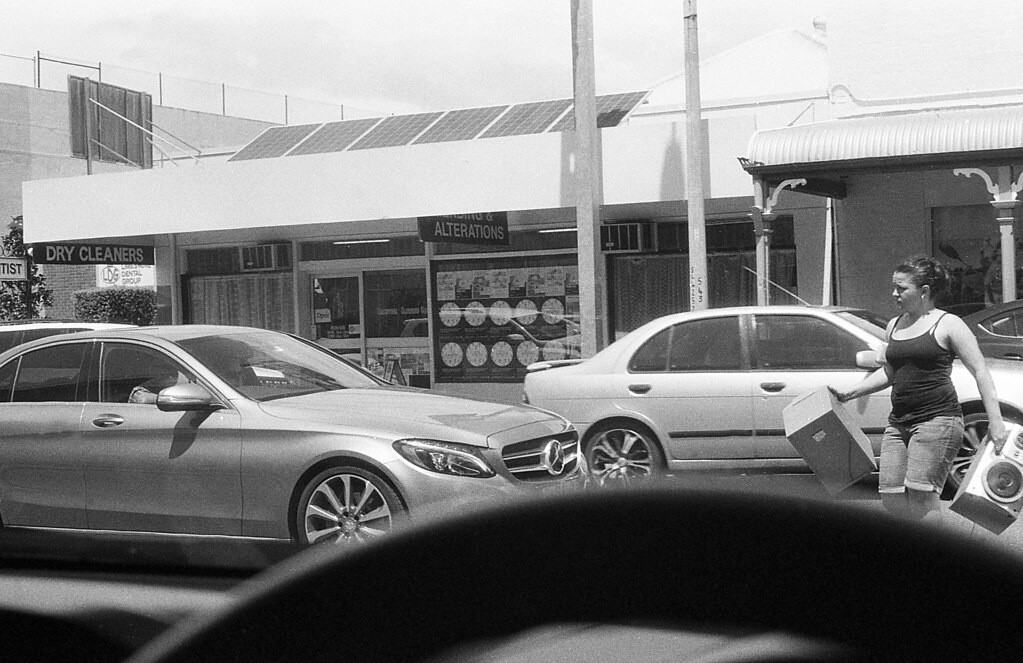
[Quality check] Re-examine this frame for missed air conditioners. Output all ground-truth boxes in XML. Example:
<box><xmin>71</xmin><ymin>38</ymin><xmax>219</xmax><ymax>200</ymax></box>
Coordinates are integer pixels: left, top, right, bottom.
<box><xmin>238</xmin><ymin>242</ymin><xmax>292</xmax><ymax>273</ymax></box>
<box><xmin>599</xmin><ymin>222</ymin><xmax>660</xmax><ymax>255</ymax></box>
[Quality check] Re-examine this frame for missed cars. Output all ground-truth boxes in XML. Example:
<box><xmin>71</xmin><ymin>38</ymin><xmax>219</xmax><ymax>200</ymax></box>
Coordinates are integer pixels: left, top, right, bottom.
<box><xmin>0</xmin><ymin>326</ymin><xmax>589</xmax><ymax>549</ymax></box>
<box><xmin>521</xmin><ymin>303</ymin><xmax>1023</xmax><ymax>494</ymax></box>
<box><xmin>960</xmin><ymin>300</ymin><xmax>1023</xmax><ymax>360</ymax></box>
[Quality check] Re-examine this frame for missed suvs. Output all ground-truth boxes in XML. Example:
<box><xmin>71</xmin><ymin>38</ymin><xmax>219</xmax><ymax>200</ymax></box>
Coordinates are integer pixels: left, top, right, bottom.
<box><xmin>0</xmin><ymin>318</ymin><xmax>292</xmax><ymax>390</ymax></box>
<box><xmin>402</xmin><ymin>307</ymin><xmax>581</xmax><ymax>359</ymax></box>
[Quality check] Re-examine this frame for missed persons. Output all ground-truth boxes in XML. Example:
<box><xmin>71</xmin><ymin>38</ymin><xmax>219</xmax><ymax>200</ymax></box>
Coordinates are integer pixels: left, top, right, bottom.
<box><xmin>127</xmin><ymin>355</ymin><xmax>195</xmax><ymax>403</ymax></box>
<box><xmin>827</xmin><ymin>254</ymin><xmax>1007</xmax><ymax>526</ymax></box>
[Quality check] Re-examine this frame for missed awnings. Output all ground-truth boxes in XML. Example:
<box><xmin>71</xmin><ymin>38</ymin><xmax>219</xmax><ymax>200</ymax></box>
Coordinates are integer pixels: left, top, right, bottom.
<box><xmin>21</xmin><ymin>112</ymin><xmax>758</xmax><ymax>246</ymax></box>
